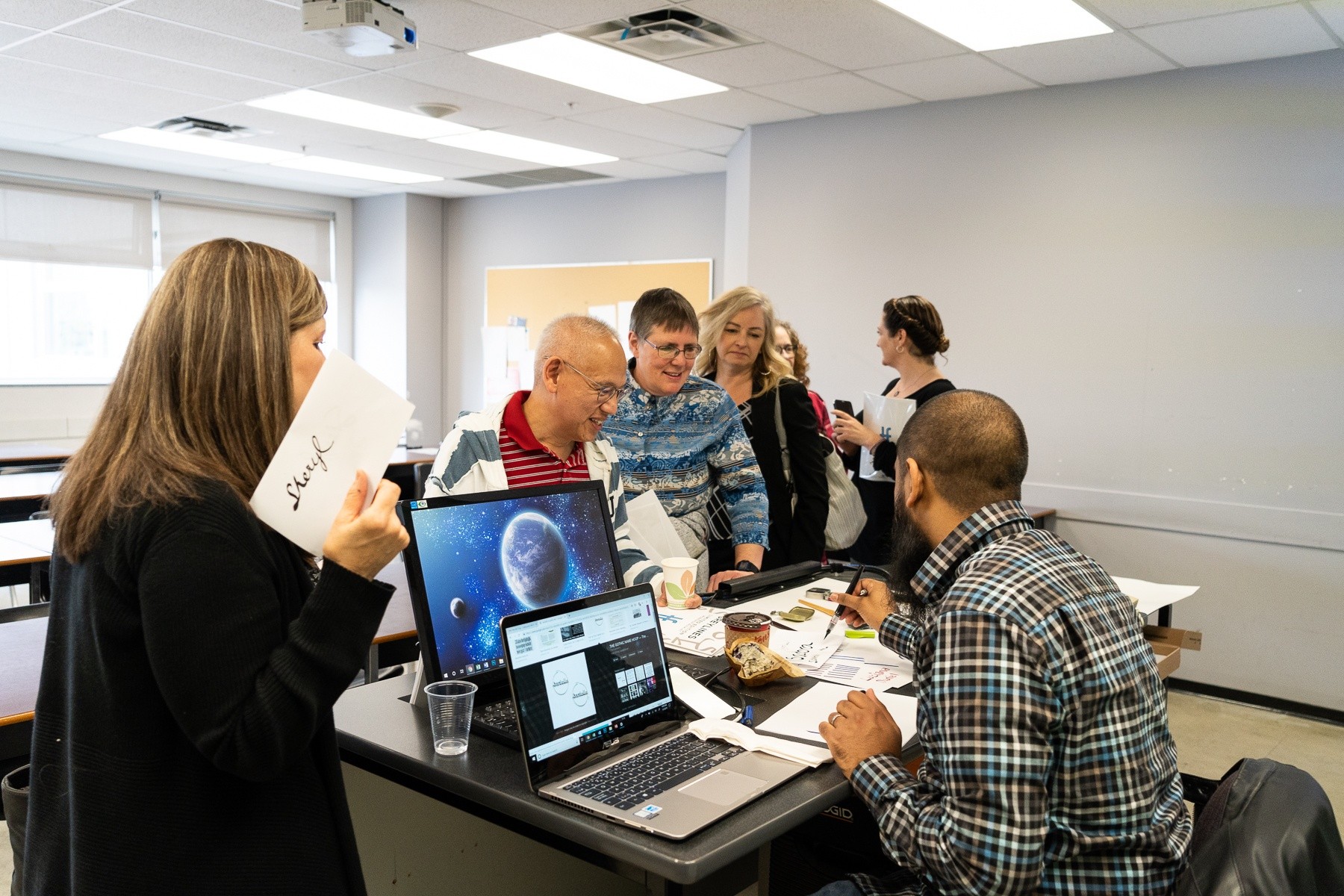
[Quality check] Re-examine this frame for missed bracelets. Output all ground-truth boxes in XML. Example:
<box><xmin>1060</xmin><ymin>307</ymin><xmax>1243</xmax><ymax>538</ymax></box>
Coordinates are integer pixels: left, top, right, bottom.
<box><xmin>869</xmin><ymin>438</ymin><xmax>886</xmax><ymax>454</ymax></box>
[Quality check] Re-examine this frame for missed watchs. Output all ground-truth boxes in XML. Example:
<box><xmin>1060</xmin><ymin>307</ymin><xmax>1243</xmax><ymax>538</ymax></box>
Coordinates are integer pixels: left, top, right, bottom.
<box><xmin>735</xmin><ymin>560</ymin><xmax>760</xmax><ymax>573</ymax></box>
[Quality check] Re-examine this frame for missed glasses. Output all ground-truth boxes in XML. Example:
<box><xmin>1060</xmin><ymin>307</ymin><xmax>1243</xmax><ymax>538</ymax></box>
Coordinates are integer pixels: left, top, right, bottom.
<box><xmin>775</xmin><ymin>344</ymin><xmax>799</xmax><ymax>359</ymax></box>
<box><xmin>543</xmin><ymin>356</ymin><xmax>630</xmax><ymax>404</ymax></box>
<box><xmin>637</xmin><ymin>333</ymin><xmax>704</xmax><ymax>359</ymax></box>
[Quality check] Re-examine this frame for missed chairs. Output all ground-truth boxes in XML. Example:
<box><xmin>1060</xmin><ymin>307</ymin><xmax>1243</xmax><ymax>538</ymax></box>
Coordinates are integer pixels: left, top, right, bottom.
<box><xmin>1173</xmin><ymin>756</ymin><xmax>1344</xmax><ymax>896</ymax></box>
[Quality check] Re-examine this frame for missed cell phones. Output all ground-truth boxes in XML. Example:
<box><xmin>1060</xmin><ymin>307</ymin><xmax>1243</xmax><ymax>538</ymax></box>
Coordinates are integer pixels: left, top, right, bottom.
<box><xmin>834</xmin><ymin>400</ymin><xmax>854</xmax><ymax>427</ymax></box>
<box><xmin>668</xmin><ymin>665</ymin><xmax>742</xmax><ymax>723</ymax></box>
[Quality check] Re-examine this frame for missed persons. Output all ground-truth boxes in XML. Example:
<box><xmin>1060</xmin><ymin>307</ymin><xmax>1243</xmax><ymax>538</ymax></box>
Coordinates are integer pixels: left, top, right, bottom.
<box><xmin>598</xmin><ymin>287</ymin><xmax>771</xmax><ymax>593</ymax></box>
<box><xmin>423</xmin><ymin>316</ymin><xmax>703</xmax><ymax>609</ymax></box>
<box><xmin>831</xmin><ymin>294</ymin><xmax>957</xmax><ymax>566</ymax></box>
<box><xmin>771</xmin><ymin>319</ymin><xmax>838</xmax><ymax>447</ymax></box>
<box><xmin>693</xmin><ymin>286</ymin><xmax>829</xmax><ymax>579</ymax></box>
<box><xmin>20</xmin><ymin>236</ymin><xmax>411</xmax><ymax>896</ymax></box>
<box><xmin>812</xmin><ymin>389</ymin><xmax>1192</xmax><ymax>896</ymax></box>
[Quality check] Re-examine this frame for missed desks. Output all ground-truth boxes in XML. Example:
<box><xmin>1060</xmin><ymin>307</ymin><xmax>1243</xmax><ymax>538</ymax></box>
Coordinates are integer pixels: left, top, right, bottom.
<box><xmin>0</xmin><ymin>449</ymin><xmax>1205</xmax><ymax>896</ymax></box>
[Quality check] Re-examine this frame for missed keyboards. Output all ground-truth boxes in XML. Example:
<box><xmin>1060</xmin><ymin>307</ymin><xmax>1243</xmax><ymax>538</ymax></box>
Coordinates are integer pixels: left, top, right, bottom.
<box><xmin>471</xmin><ymin>658</ymin><xmax>717</xmax><ymax>751</ymax></box>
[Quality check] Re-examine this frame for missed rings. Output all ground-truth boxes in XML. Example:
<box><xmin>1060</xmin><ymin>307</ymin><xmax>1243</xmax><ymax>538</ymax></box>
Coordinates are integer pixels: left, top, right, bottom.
<box><xmin>831</xmin><ymin>714</ymin><xmax>842</xmax><ymax>725</ymax></box>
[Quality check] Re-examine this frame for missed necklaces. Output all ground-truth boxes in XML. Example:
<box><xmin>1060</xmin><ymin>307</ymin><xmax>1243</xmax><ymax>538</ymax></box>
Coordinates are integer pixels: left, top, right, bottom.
<box><xmin>893</xmin><ymin>367</ymin><xmax>936</xmax><ymax>397</ymax></box>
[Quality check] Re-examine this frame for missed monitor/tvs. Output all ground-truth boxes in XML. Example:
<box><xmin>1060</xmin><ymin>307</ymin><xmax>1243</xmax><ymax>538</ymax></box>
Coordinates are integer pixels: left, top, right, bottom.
<box><xmin>395</xmin><ymin>477</ymin><xmax>626</xmax><ymax>706</ymax></box>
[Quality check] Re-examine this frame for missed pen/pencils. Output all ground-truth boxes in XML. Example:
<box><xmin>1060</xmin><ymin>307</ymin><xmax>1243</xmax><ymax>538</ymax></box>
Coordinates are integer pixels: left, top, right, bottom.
<box><xmin>823</xmin><ymin>563</ymin><xmax>866</xmax><ymax>640</ymax></box>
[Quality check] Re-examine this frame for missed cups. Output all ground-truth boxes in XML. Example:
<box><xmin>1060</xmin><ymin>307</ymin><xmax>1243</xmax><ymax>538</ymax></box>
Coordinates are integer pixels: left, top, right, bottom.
<box><xmin>722</xmin><ymin>613</ymin><xmax>771</xmax><ymax>669</ymax></box>
<box><xmin>661</xmin><ymin>557</ymin><xmax>699</xmax><ymax>611</ymax></box>
<box><xmin>424</xmin><ymin>681</ymin><xmax>478</xmax><ymax>755</ymax></box>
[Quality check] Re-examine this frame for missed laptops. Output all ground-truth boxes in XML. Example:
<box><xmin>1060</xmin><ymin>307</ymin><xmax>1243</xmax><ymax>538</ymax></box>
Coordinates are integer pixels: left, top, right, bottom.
<box><xmin>499</xmin><ymin>580</ymin><xmax>810</xmax><ymax>840</ymax></box>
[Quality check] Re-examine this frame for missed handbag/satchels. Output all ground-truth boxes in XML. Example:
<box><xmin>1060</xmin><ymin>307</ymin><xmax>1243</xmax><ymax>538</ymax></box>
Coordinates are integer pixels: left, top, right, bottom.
<box><xmin>818</xmin><ymin>433</ymin><xmax>867</xmax><ymax>549</ymax></box>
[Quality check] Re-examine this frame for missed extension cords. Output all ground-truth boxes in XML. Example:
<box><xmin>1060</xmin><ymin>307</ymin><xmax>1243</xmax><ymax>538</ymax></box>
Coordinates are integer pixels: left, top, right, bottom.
<box><xmin>718</xmin><ymin>561</ymin><xmax>821</xmax><ymax>595</ymax></box>
